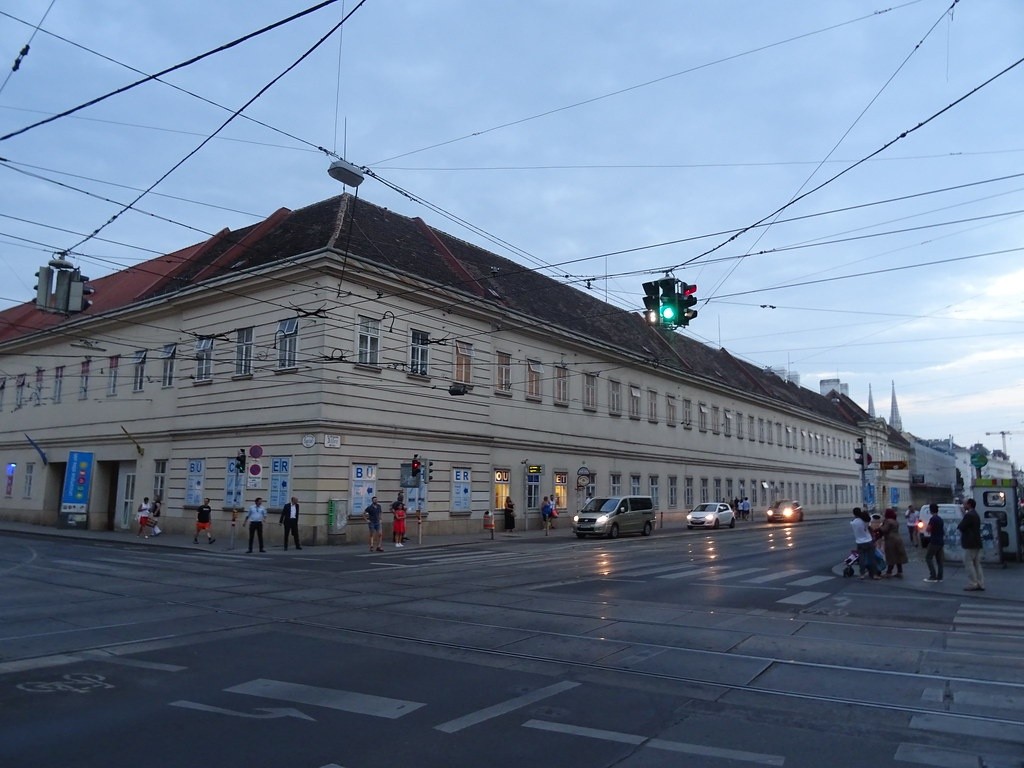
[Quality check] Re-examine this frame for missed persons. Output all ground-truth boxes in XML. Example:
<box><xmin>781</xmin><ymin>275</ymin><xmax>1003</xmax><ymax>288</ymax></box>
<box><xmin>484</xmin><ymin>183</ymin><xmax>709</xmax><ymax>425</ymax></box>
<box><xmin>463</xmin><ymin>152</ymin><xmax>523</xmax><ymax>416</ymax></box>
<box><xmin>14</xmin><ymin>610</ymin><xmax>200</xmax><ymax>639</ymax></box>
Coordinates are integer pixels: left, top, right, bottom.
<box><xmin>851</xmin><ymin>507</ymin><xmax>908</xmax><ymax>579</ymax></box>
<box><xmin>958</xmin><ymin>499</ymin><xmax>984</xmax><ymax>590</ymax></box>
<box><xmin>363</xmin><ymin>496</ymin><xmax>384</xmax><ymax>551</ymax></box>
<box><xmin>243</xmin><ymin>498</ymin><xmax>266</xmax><ymax>553</ymax></box>
<box><xmin>389</xmin><ymin>492</ymin><xmax>410</xmax><ymax>547</ymax></box>
<box><xmin>193</xmin><ymin>498</ymin><xmax>216</xmax><ymax>544</ymax></box>
<box><xmin>722</xmin><ymin>497</ymin><xmax>750</xmax><ymax>521</ymax></box>
<box><xmin>585</xmin><ymin>493</ymin><xmax>592</xmax><ymax>507</ymax></box>
<box><xmin>924</xmin><ymin>503</ymin><xmax>944</xmax><ymax>582</ymax></box>
<box><xmin>541</xmin><ymin>495</ymin><xmax>556</xmax><ymax>530</ymax></box>
<box><xmin>505</xmin><ymin>497</ymin><xmax>515</xmax><ymax>532</ymax></box>
<box><xmin>279</xmin><ymin>496</ymin><xmax>302</xmax><ymax>550</ymax></box>
<box><xmin>905</xmin><ymin>505</ymin><xmax>919</xmax><ymax>547</ymax></box>
<box><xmin>136</xmin><ymin>496</ymin><xmax>162</xmax><ymax>538</ymax></box>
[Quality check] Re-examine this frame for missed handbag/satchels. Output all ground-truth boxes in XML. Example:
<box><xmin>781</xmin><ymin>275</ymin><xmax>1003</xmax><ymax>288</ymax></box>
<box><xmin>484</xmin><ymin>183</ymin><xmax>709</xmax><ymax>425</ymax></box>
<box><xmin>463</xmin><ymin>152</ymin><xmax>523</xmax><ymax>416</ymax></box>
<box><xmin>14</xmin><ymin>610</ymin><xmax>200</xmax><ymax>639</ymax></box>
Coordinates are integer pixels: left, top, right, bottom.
<box><xmin>135</xmin><ymin>509</ymin><xmax>141</xmax><ymax>524</ymax></box>
<box><xmin>511</xmin><ymin>505</ymin><xmax>518</xmax><ymax>517</ymax></box>
<box><xmin>147</xmin><ymin>516</ymin><xmax>158</xmax><ymax>528</ymax></box>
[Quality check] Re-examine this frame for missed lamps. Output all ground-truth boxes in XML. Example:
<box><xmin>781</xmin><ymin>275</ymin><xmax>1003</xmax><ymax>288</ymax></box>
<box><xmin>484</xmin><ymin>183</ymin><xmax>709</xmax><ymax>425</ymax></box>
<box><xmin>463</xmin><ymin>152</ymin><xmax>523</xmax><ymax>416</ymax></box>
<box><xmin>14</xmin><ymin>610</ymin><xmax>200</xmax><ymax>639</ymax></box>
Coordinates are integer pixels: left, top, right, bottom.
<box><xmin>447</xmin><ymin>384</ymin><xmax>467</xmax><ymax>395</ymax></box>
<box><xmin>328</xmin><ymin>161</ymin><xmax>365</xmax><ymax>187</ymax></box>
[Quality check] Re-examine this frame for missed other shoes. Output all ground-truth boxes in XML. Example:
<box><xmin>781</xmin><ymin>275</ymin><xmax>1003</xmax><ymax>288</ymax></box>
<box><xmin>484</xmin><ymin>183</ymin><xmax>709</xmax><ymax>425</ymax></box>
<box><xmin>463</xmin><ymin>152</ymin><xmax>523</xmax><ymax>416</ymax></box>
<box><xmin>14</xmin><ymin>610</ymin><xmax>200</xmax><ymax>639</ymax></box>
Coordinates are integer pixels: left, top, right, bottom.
<box><xmin>395</xmin><ymin>543</ymin><xmax>401</xmax><ymax>547</ymax></box>
<box><xmin>893</xmin><ymin>572</ymin><xmax>902</xmax><ymax>578</ymax></box>
<box><xmin>284</xmin><ymin>547</ymin><xmax>302</xmax><ymax>551</ymax></box>
<box><xmin>209</xmin><ymin>539</ymin><xmax>215</xmax><ymax>544</ymax></box>
<box><xmin>399</xmin><ymin>543</ymin><xmax>403</xmax><ymax>547</ymax></box>
<box><xmin>192</xmin><ymin>538</ymin><xmax>198</xmax><ymax>544</ymax></box>
<box><xmin>937</xmin><ymin>575</ymin><xmax>944</xmax><ymax>582</ymax></box>
<box><xmin>964</xmin><ymin>583</ymin><xmax>979</xmax><ymax>591</ymax></box>
<box><xmin>861</xmin><ymin>574</ymin><xmax>866</xmax><ymax>579</ymax></box>
<box><xmin>874</xmin><ymin>576</ymin><xmax>881</xmax><ymax>580</ymax></box>
<box><xmin>136</xmin><ymin>532</ymin><xmax>162</xmax><ymax>539</ymax></box>
<box><xmin>923</xmin><ymin>576</ymin><xmax>938</xmax><ymax>582</ymax></box>
<box><xmin>978</xmin><ymin>586</ymin><xmax>985</xmax><ymax>590</ymax></box>
<box><xmin>370</xmin><ymin>546</ymin><xmax>374</xmax><ymax>552</ymax></box>
<box><xmin>259</xmin><ymin>550</ymin><xmax>266</xmax><ymax>552</ymax></box>
<box><xmin>376</xmin><ymin>547</ymin><xmax>383</xmax><ymax>551</ymax></box>
<box><xmin>245</xmin><ymin>551</ymin><xmax>252</xmax><ymax>553</ymax></box>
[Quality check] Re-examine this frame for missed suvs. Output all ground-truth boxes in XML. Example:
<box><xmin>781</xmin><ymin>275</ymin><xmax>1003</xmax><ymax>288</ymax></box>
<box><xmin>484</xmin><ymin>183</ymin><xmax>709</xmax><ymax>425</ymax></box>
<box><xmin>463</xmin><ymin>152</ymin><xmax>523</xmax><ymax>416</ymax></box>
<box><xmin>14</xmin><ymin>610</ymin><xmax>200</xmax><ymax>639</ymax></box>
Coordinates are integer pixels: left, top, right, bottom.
<box><xmin>571</xmin><ymin>496</ymin><xmax>656</xmax><ymax>540</ymax></box>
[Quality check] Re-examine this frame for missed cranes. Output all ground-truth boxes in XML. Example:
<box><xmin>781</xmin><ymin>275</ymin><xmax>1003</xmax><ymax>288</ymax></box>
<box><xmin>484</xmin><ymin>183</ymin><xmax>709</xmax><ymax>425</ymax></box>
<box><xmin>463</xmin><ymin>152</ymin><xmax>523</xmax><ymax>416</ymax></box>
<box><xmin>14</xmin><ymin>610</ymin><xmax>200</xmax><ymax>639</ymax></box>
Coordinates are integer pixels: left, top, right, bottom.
<box><xmin>985</xmin><ymin>430</ymin><xmax>1024</xmax><ymax>462</ymax></box>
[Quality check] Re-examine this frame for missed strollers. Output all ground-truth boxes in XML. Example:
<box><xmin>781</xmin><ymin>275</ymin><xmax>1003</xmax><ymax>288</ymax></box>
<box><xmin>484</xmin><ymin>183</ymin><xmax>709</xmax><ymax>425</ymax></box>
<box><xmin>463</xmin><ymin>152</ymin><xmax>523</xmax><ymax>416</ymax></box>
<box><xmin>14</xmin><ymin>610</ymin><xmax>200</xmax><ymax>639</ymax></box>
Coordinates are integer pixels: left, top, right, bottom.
<box><xmin>841</xmin><ymin>525</ymin><xmax>885</xmax><ymax>579</ymax></box>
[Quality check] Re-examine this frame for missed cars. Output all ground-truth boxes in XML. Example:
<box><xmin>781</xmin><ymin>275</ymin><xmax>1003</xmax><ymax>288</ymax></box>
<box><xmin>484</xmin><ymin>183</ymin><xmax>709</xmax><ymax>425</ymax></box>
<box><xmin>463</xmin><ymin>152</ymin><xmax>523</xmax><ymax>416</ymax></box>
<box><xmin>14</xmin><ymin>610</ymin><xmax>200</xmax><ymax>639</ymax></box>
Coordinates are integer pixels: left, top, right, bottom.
<box><xmin>766</xmin><ymin>498</ymin><xmax>804</xmax><ymax>524</ymax></box>
<box><xmin>914</xmin><ymin>502</ymin><xmax>968</xmax><ymax>549</ymax></box>
<box><xmin>685</xmin><ymin>502</ymin><xmax>737</xmax><ymax>530</ymax></box>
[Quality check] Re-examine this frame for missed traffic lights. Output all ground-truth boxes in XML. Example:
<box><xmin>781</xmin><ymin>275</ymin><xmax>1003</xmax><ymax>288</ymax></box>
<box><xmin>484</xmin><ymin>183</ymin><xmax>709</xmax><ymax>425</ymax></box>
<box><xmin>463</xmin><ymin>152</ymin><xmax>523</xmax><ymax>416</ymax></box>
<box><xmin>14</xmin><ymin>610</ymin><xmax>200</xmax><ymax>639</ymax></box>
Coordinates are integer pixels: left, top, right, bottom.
<box><xmin>67</xmin><ymin>269</ymin><xmax>95</xmax><ymax>314</ymax></box>
<box><xmin>677</xmin><ymin>281</ymin><xmax>698</xmax><ymax>327</ymax></box>
<box><xmin>32</xmin><ymin>266</ymin><xmax>55</xmax><ymax>312</ymax></box>
<box><xmin>411</xmin><ymin>458</ymin><xmax>421</xmax><ymax>477</ymax></box>
<box><xmin>642</xmin><ymin>280</ymin><xmax>661</xmax><ymax>326</ymax></box>
<box><xmin>235</xmin><ymin>454</ymin><xmax>246</xmax><ymax>473</ymax></box>
<box><xmin>854</xmin><ymin>444</ymin><xmax>863</xmax><ymax>465</ymax></box>
<box><xmin>424</xmin><ymin>459</ymin><xmax>433</xmax><ymax>484</ymax></box>
<box><xmin>657</xmin><ymin>277</ymin><xmax>677</xmax><ymax>328</ymax></box>
